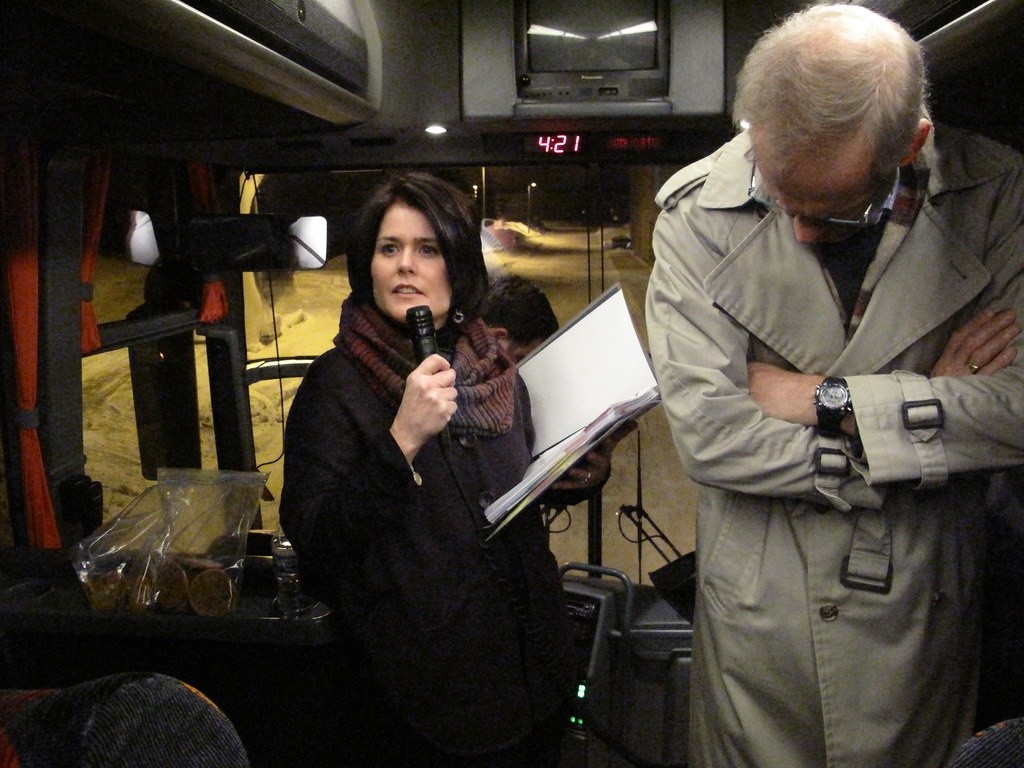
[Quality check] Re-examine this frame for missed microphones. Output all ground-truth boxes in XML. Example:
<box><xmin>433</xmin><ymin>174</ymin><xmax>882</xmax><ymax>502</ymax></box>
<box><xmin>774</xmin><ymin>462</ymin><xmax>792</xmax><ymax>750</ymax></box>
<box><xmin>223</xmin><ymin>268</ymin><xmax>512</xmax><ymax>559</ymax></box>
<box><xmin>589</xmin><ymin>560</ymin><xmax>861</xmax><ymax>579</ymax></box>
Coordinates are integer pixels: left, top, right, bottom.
<box><xmin>407</xmin><ymin>306</ymin><xmax>452</xmax><ymax>451</ymax></box>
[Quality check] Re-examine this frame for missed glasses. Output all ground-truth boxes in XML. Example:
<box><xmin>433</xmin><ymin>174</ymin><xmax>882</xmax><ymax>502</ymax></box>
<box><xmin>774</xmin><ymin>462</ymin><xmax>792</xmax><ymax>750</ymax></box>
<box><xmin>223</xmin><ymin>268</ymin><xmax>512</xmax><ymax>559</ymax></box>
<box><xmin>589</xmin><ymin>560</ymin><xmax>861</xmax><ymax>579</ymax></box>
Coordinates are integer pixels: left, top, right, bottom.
<box><xmin>748</xmin><ymin>159</ymin><xmax>900</xmax><ymax>229</ymax></box>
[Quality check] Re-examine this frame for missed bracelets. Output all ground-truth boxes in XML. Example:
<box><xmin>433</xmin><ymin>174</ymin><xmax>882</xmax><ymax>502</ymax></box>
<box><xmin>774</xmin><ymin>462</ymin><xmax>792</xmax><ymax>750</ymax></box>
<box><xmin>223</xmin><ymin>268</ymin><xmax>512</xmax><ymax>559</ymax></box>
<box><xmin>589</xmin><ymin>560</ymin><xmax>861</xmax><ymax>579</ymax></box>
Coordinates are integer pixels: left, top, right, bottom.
<box><xmin>409</xmin><ymin>463</ymin><xmax>423</xmax><ymax>486</ymax></box>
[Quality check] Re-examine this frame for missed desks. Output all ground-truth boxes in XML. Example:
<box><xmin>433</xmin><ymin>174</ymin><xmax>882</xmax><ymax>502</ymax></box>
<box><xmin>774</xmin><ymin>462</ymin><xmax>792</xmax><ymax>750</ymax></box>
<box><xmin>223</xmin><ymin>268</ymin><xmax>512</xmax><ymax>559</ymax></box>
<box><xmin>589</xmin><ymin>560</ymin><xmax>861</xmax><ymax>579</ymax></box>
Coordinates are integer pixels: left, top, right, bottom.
<box><xmin>0</xmin><ymin>553</ymin><xmax>347</xmax><ymax>646</ymax></box>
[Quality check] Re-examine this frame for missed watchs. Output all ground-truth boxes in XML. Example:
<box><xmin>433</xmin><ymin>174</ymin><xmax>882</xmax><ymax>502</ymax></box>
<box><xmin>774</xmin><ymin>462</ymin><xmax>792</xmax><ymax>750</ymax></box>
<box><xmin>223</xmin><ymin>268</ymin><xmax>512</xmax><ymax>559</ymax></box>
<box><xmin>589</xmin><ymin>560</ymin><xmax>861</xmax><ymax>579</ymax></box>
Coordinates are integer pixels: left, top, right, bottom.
<box><xmin>812</xmin><ymin>375</ymin><xmax>853</xmax><ymax>433</ymax></box>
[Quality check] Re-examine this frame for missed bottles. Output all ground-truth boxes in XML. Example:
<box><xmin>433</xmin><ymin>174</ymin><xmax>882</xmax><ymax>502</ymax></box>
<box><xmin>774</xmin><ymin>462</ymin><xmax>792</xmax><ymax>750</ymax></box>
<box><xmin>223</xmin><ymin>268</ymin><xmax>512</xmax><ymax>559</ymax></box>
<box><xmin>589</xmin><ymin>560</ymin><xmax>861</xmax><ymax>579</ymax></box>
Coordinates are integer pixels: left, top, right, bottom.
<box><xmin>271</xmin><ymin>520</ymin><xmax>300</xmax><ymax>613</ymax></box>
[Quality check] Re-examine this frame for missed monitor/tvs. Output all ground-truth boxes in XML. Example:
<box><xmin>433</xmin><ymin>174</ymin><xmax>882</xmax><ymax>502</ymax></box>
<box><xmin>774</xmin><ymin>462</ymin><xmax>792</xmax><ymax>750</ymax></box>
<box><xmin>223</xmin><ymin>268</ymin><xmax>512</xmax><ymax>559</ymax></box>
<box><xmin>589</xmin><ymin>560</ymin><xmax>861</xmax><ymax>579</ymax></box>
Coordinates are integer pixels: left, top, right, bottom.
<box><xmin>512</xmin><ymin>0</ymin><xmax>671</xmax><ymax>101</ymax></box>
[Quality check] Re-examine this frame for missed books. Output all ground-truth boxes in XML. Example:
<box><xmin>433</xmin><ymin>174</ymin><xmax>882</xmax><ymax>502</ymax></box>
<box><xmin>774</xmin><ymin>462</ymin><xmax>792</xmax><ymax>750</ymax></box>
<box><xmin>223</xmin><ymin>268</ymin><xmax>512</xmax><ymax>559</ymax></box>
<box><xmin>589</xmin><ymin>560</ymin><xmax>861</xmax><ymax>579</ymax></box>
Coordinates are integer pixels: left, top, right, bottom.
<box><xmin>483</xmin><ymin>281</ymin><xmax>660</xmax><ymax>544</ymax></box>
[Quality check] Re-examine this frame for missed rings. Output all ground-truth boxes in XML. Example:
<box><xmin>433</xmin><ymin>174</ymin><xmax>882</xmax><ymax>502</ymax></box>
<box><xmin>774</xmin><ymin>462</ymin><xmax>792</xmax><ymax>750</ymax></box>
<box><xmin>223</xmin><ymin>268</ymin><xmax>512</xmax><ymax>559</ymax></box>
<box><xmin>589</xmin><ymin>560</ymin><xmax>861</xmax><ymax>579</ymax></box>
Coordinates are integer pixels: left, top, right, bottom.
<box><xmin>581</xmin><ymin>472</ymin><xmax>591</xmax><ymax>483</ymax></box>
<box><xmin>967</xmin><ymin>362</ymin><xmax>980</xmax><ymax>373</ymax></box>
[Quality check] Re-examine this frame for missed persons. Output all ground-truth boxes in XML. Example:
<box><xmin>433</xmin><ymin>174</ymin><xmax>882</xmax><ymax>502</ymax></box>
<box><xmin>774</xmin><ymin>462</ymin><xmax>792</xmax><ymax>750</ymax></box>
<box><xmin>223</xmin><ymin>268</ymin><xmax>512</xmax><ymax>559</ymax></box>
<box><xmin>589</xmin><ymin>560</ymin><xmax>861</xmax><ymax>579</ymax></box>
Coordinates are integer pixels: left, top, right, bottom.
<box><xmin>274</xmin><ymin>174</ymin><xmax>639</xmax><ymax>768</ymax></box>
<box><xmin>644</xmin><ymin>5</ymin><xmax>1024</xmax><ymax>768</ymax></box>
<box><xmin>478</xmin><ymin>274</ymin><xmax>560</xmax><ymax>367</ymax></box>
